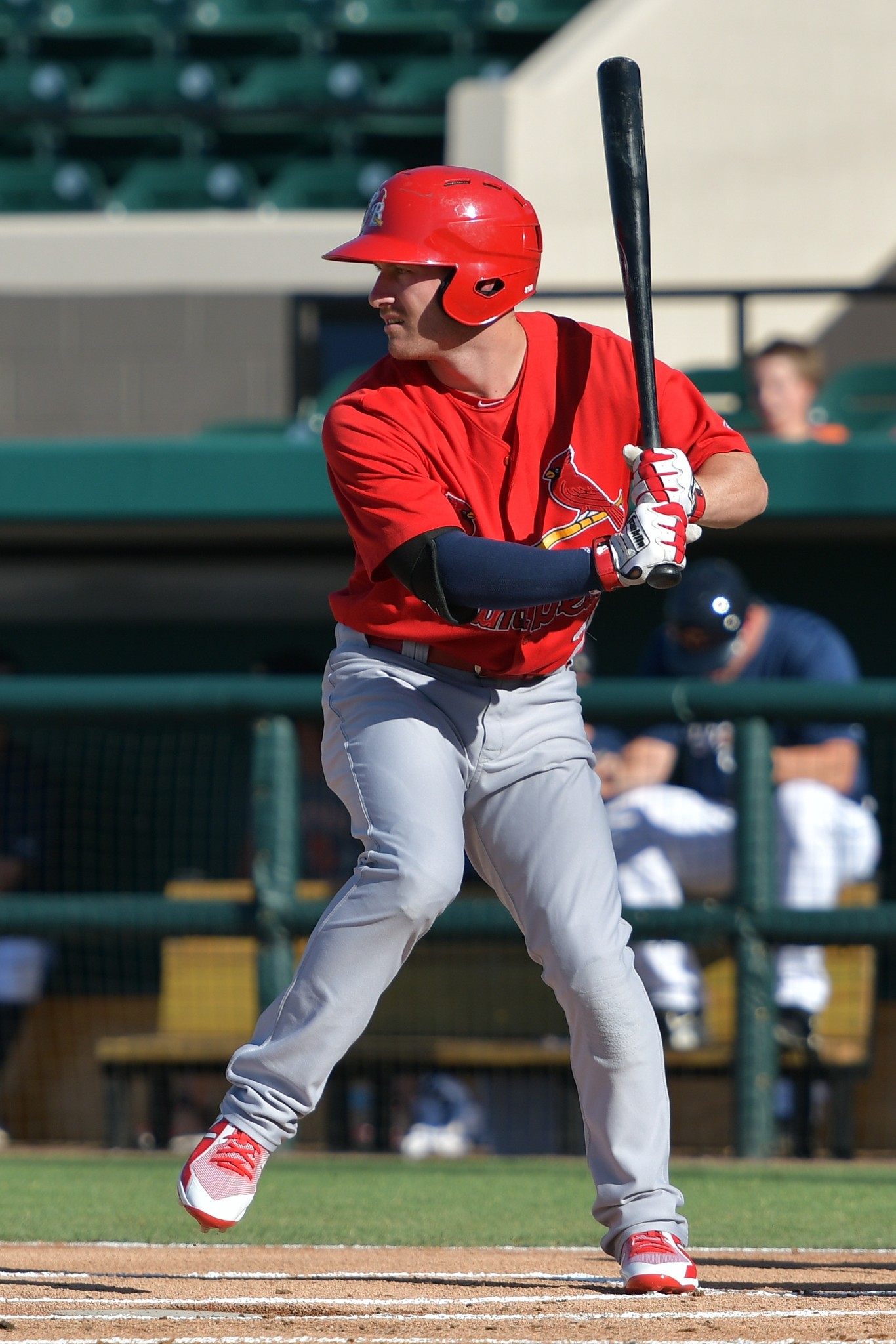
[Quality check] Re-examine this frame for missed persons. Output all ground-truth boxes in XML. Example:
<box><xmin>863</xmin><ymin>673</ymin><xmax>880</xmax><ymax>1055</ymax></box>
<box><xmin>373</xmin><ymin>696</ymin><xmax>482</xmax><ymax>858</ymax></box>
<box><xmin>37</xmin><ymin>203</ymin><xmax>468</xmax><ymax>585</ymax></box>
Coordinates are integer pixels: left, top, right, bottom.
<box><xmin>0</xmin><ymin>840</ymin><xmax>44</xmax><ymax>1144</ymax></box>
<box><xmin>594</xmin><ymin>552</ymin><xmax>881</xmax><ymax>1060</ymax></box>
<box><xmin>180</xmin><ymin>168</ymin><xmax>768</xmax><ymax>1295</ymax></box>
<box><xmin>738</xmin><ymin>339</ymin><xmax>846</xmax><ymax>446</ymax></box>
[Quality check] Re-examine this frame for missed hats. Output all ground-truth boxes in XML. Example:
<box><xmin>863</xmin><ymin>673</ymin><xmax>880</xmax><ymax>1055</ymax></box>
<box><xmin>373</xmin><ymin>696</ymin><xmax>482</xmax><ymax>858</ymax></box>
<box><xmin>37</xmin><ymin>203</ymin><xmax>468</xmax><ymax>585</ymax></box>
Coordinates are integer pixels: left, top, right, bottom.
<box><xmin>661</xmin><ymin>559</ymin><xmax>753</xmax><ymax>676</ymax></box>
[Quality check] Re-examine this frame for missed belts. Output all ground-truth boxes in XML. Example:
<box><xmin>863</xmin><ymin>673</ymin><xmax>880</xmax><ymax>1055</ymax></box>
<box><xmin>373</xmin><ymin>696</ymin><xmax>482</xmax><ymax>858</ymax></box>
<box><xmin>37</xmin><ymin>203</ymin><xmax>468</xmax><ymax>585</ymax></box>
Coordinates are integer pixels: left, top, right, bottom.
<box><xmin>361</xmin><ymin>633</ymin><xmax>539</xmax><ymax>682</ymax></box>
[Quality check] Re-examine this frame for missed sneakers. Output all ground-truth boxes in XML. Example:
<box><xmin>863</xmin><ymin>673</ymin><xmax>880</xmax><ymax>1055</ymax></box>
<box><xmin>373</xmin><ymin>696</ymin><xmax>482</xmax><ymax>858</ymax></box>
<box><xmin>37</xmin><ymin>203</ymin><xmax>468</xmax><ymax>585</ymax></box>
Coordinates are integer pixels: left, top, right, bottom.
<box><xmin>175</xmin><ymin>1113</ymin><xmax>271</xmax><ymax>1232</ymax></box>
<box><xmin>622</xmin><ymin>1231</ymin><xmax>699</xmax><ymax>1294</ymax></box>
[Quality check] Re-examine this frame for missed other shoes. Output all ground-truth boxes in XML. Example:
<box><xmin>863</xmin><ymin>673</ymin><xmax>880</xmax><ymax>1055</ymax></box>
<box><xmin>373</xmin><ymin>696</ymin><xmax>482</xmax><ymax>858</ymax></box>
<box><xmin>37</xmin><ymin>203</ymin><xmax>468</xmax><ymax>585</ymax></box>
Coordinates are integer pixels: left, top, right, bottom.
<box><xmin>656</xmin><ymin>1009</ymin><xmax>706</xmax><ymax>1049</ymax></box>
<box><xmin>773</xmin><ymin>1014</ymin><xmax>813</xmax><ymax>1071</ymax></box>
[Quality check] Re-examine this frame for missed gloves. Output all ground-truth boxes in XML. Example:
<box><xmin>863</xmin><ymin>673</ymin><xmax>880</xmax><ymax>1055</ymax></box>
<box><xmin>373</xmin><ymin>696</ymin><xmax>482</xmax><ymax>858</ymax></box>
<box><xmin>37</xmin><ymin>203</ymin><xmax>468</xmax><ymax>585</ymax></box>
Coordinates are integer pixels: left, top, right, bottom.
<box><xmin>591</xmin><ymin>501</ymin><xmax>702</xmax><ymax>595</ymax></box>
<box><xmin>621</xmin><ymin>444</ymin><xmax>706</xmax><ymax>525</ymax></box>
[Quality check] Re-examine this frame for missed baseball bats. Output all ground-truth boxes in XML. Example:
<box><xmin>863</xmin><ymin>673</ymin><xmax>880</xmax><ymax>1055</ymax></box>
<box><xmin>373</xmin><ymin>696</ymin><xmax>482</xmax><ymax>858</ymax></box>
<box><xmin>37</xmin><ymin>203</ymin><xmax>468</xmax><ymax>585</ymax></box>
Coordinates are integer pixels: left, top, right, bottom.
<box><xmin>598</xmin><ymin>54</ymin><xmax>687</xmax><ymax>589</ymax></box>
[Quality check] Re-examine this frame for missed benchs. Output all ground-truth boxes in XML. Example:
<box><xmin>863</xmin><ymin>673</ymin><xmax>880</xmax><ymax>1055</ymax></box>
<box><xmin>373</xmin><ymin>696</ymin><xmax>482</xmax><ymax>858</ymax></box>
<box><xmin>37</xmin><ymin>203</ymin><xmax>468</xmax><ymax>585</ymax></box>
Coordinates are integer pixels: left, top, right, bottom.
<box><xmin>95</xmin><ymin>877</ymin><xmax>876</xmax><ymax>1159</ymax></box>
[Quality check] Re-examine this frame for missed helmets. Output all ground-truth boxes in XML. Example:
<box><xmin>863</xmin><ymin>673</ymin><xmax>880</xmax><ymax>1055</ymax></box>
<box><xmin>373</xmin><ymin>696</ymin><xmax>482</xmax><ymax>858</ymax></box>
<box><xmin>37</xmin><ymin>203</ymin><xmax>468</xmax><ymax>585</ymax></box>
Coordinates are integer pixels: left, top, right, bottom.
<box><xmin>325</xmin><ymin>166</ymin><xmax>542</xmax><ymax>328</ymax></box>
